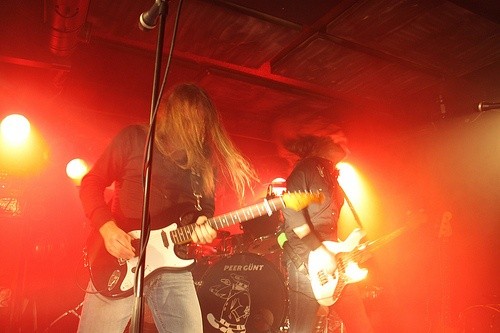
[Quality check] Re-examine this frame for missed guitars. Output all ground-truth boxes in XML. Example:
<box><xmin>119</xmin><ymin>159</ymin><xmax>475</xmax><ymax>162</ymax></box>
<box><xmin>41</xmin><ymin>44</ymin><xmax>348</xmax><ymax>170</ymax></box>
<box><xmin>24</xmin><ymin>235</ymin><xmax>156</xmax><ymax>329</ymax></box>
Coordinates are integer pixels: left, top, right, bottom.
<box><xmin>88</xmin><ymin>189</ymin><xmax>326</xmax><ymax>300</ymax></box>
<box><xmin>308</xmin><ymin>209</ymin><xmax>427</xmax><ymax>307</ymax></box>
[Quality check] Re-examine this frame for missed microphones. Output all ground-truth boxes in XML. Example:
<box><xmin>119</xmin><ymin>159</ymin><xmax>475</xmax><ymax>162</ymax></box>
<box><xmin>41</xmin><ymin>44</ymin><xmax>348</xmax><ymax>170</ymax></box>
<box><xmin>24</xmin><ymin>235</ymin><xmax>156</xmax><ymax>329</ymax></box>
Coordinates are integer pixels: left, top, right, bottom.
<box><xmin>475</xmin><ymin>102</ymin><xmax>500</xmax><ymax>112</ymax></box>
<box><xmin>138</xmin><ymin>0</ymin><xmax>162</xmax><ymax>31</ymax></box>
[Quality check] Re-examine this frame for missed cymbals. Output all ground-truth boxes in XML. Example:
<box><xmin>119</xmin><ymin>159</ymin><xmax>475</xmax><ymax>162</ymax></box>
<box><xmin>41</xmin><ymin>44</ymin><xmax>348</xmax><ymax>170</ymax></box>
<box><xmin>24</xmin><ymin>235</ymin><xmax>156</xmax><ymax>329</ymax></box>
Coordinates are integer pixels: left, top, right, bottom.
<box><xmin>262</xmin><ymin>182</ymin><xmax>287</xmax><ymax>188</ymax></box>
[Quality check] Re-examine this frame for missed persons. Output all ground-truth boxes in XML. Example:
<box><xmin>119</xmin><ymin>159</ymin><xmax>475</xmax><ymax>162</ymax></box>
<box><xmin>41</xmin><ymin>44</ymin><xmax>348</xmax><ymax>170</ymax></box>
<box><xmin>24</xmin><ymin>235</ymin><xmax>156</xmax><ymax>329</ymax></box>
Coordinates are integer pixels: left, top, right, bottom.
<box><xmin>77</xmin><ymin>84</ymin><xmax>261</xmax><ymax>333</ymax></box>
<box><xmin>283</xmin><ymin>135</ymin><xmax>374</xmax><ymax>333</ymax></box>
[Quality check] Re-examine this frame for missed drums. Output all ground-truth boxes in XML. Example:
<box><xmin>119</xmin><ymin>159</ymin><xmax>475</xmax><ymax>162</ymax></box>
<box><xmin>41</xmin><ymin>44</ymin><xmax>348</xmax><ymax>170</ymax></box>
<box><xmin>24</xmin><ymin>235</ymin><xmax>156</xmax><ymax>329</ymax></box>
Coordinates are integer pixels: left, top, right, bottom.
<box><xmin>186</xmin><ymin>230</ymin><xmax>224</xmax><ymax>257</ymax></box>
<box><xmin>239</xmin><ymin>201</ymin><xmax>281</xmax><ymax>234</ymax></box>
<box><xmin>197</xmin><ymin>251</ymin><xmax>289</xmax><ymax>333</ymax></box>
<box><xmin>246</xmin><ymin>234</ymin><xmax>287</xmax><ymax>282</ymax></box>
